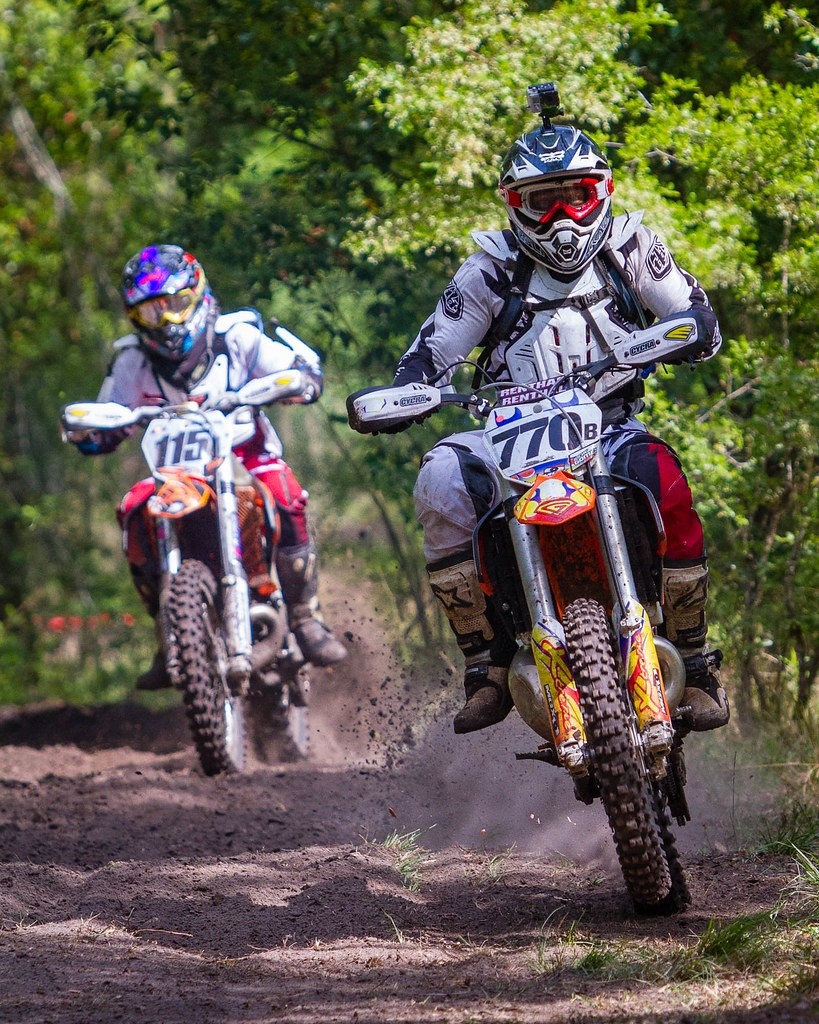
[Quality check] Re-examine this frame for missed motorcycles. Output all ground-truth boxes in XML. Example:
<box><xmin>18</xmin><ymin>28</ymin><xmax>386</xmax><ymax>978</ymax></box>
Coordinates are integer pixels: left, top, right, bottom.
<box><xmin>62</xmin><ymin>369</ymin><xmax>314</xmax><ymax>777</ymax></box>
<box><xmin>344</xmin><ymin>309</ymin><xmax>711</xmax><ymax>906</ymax></box>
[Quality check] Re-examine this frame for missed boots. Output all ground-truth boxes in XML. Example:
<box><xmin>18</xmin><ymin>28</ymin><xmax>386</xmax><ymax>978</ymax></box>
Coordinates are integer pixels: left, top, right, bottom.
<box><xmin>662</xmin><ymin>555</ymin><xmax>732</xmax><ymax>732</ymax></box>
<box><xmin>134</xmin><ymin>574</ymin><xmax>173</xmax><ymax>690</ymax></box>
<box><xmin>273</xmin><ymin>539</ymin><xmax>349</xmax><ymax>669</ymax></box>
<box><xmin>425</xmin><ymin>547</ymin><xmax>517</xmax><ymax>732</ymax></box>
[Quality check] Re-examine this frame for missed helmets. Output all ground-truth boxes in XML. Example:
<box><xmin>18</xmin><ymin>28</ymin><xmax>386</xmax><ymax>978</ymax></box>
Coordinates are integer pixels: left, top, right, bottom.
<box><xmin>502</xmin><ymin>124</ymin><xmax>614</xmax><ymax>276</ymax></box>
<box><xmin>119</xmin><ymin>244</ymin><xmax>212</xmax><ymax>360</ymax></box>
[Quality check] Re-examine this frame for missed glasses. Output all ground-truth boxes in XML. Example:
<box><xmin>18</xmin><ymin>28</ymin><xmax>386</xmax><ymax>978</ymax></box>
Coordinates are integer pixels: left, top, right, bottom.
<box><xmin>128</xmin><ymin>290</ymin><xmax>199</xmax><ymax>329</ymax></box>
<box><xmin>513</xmin><ymin>178</ymin><xmax>608</xmax><ymax>222</ymax></box>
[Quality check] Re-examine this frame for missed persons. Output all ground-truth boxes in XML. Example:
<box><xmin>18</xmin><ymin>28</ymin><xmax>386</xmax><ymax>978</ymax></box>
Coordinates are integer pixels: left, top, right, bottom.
<box><xmin>371</xmin><ymin>83</ymin><xmax>729</xmax><ymax>734</ymax></box>
<box><xmin>64</xmin><ymin>245</ymin><xmax>348</xmax><ymax>691</ymax></box>
